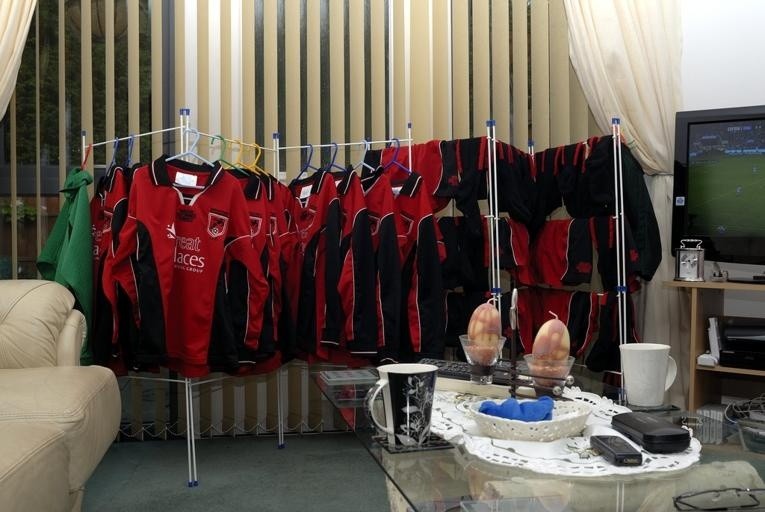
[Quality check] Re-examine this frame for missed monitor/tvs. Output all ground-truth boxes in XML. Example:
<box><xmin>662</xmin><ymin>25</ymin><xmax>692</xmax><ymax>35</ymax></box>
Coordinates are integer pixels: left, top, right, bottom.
<box><xmin>671</xmin><ymin>105</ymin><xmax>765</xmax><ymax>284</ymax></box>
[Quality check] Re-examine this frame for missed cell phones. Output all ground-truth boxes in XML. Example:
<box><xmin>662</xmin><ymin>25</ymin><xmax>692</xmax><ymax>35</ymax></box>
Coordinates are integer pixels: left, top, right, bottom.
<box><xmin>590</xmin><ymin>435</ymin><xmax>642</xmax><ymax>466</ymax></box>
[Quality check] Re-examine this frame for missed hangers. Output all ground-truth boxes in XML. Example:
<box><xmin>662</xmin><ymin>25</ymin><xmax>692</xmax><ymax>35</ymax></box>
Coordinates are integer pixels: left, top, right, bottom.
<box><xmin>78</xmin><ymin>129</ymin><xmax>138</xmax><ymax>186</ymax></box>
<box><xmin>291</xmin><ymin>137</ymin><xmax>411</xmax><ymax>185</ymax></box>
<box><xmin>161</xmin><ymin>129</ymin><xmax>271</xmax><ymax>186</ymax></box>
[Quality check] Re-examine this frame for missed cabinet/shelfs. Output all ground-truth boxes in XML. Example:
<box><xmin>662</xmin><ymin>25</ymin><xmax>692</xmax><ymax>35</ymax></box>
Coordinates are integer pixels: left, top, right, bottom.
<box><xmin>662</xmin><ymin>278</ymin><xmax>765</xmax><ymax>434</ymax></box>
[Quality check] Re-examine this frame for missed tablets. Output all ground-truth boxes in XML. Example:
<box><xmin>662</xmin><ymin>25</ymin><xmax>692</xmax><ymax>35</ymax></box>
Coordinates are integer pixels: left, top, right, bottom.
<box><xmin>319</xmin><ymin>369</ymin><xmax>380</xmax><ymax>382</ymax></box>
<box><xmin>320</xmin><ymin>374</ymin><xmax>376</xmax><ymax>385</ymax></box>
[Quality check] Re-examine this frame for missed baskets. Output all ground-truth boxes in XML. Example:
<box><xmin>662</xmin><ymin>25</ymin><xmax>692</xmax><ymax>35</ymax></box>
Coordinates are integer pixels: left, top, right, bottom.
<box><xmin>470</xmin><ymin>399</ymin><xmax>593</xmax><ymax>442</ymax></box>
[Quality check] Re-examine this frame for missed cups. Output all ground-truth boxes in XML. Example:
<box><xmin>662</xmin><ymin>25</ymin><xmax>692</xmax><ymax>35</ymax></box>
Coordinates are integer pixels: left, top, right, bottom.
<box><xmin>361</xmin><ymin>364</ymin><xmax>441</xmax><ymax>450</ymax></box>
<box><xmin>459</xmin><ymin>333</ymin><xmax>508</xmax><ymax>387</ymax></box>
<box><xmin>519</xmin><ymin>354</ymin><xmax>575</xmax><ymax>402</ymax></box>
<box><xmin>617</xmin><ymin>339</ymin><xmax>679</xmax><ymax>412</ymax></box>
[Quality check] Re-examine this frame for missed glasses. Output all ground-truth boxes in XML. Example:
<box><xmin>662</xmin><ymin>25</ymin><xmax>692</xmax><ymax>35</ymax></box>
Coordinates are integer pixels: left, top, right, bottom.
<box><xmin>672</xmin><ymin>488</ymin><xmax>765</xmax><ymax>512</ymax></box>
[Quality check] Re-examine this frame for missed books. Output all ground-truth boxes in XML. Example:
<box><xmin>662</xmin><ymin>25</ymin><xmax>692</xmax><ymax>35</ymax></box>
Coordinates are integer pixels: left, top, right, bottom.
<box><xmin>696</xmin><ymin>405</ymin><xmax>727</xmax><ymax>446</ymax></box>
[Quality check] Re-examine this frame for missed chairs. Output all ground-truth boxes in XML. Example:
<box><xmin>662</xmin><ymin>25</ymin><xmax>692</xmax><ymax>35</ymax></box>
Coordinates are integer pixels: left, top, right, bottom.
<box><xmin>0</xmin><ymin>280</ymin><xmax>124</xmax><ymax>512</ymax></box>
